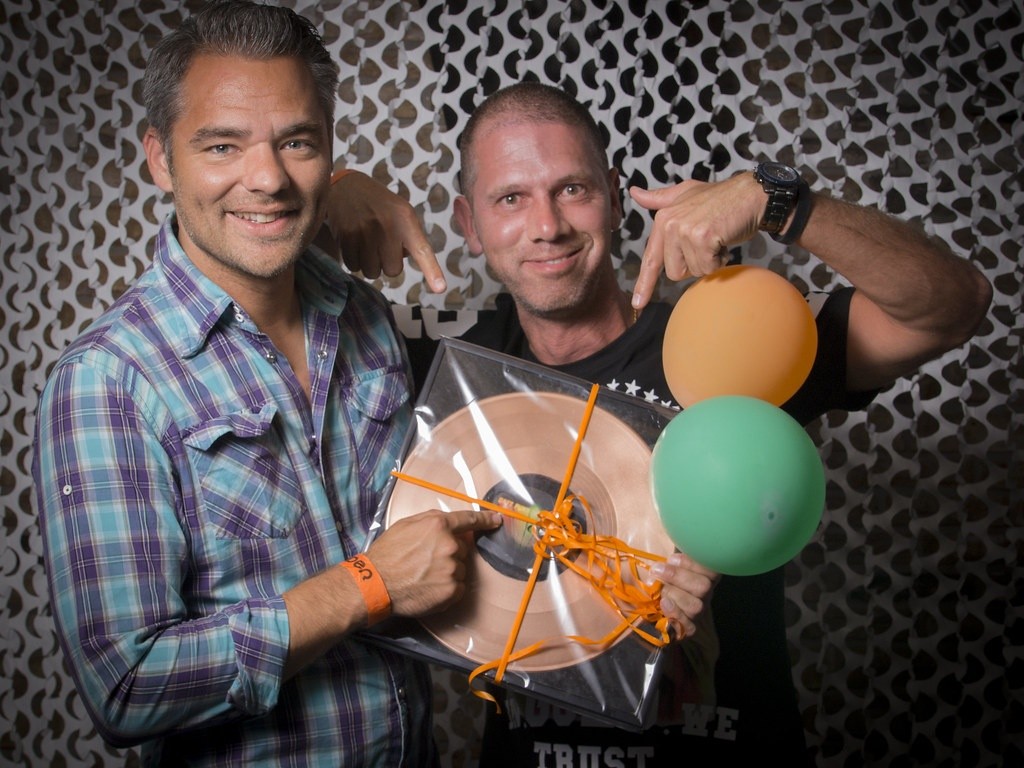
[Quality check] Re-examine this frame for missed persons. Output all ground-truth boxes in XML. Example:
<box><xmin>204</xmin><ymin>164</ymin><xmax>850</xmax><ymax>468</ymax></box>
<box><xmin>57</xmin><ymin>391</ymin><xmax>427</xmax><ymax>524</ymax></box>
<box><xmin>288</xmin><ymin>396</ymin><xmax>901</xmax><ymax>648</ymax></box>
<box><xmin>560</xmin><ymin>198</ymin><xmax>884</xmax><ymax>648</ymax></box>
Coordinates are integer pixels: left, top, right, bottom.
<box><xmin>309</xmin><ymin>82</ymin><xmax>993</xmax><ymax>768</ymax></box>
<box><xmin>31</xmin><ymin>0</ymin><xmax>722</xmax><ymax>768</ymax></box>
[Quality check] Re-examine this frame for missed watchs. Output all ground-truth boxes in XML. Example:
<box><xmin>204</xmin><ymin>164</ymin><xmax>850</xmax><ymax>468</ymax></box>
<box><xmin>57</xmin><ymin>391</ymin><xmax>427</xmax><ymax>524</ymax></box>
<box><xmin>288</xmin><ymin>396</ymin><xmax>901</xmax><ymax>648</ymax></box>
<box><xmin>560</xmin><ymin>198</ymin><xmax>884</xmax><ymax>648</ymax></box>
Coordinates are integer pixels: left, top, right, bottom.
<box><xmin>753</xmin><ymin>161</ymin><xmax>800</xmax><ymax>234</ymax></box>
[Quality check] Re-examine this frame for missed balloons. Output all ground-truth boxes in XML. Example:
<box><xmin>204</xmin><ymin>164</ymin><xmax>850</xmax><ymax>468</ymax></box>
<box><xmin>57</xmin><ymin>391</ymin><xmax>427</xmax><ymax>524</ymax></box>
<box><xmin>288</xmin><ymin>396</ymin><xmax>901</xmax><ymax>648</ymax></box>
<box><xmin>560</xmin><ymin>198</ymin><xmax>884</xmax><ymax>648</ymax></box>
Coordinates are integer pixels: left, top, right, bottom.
<box><xmin>662</xmin><ymin>263</ymin><xmax>818</xmax><ymax>410</ymax></box>
<box><xmin>649</xmin><ymin>397</ymin><xmax>827</xmax><ymax>578</ymax></box>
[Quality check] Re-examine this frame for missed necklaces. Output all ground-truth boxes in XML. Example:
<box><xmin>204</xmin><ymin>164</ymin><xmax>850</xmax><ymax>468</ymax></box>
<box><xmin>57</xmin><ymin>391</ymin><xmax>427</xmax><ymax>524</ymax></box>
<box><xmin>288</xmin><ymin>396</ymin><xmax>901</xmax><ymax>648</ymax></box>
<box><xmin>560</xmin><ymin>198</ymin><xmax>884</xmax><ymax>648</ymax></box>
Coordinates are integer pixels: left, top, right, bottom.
<box><xmin>632</xmin><ymin>309</ymin><xmax>638</xmax><ymax>324</ymax></box>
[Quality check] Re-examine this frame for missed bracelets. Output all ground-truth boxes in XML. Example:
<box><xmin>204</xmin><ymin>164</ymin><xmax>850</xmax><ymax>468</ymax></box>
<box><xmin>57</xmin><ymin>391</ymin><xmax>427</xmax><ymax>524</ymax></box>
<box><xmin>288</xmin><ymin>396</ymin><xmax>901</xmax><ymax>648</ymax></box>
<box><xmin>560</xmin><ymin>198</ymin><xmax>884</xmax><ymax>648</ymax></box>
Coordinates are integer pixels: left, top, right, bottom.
<box><xmin>768</xmin><ymin>179</ymin><xmax>811</xmax><ymax>245</ymax></box>
<box><xmin>343</xmin><ymin>555</ymin><xmax>393</xmax><ymax>628</ymax></box>
<box><xmin>329</xmin><ymin>169</ymin><xmax>358</xmax><ymax>184</ymax></box>
